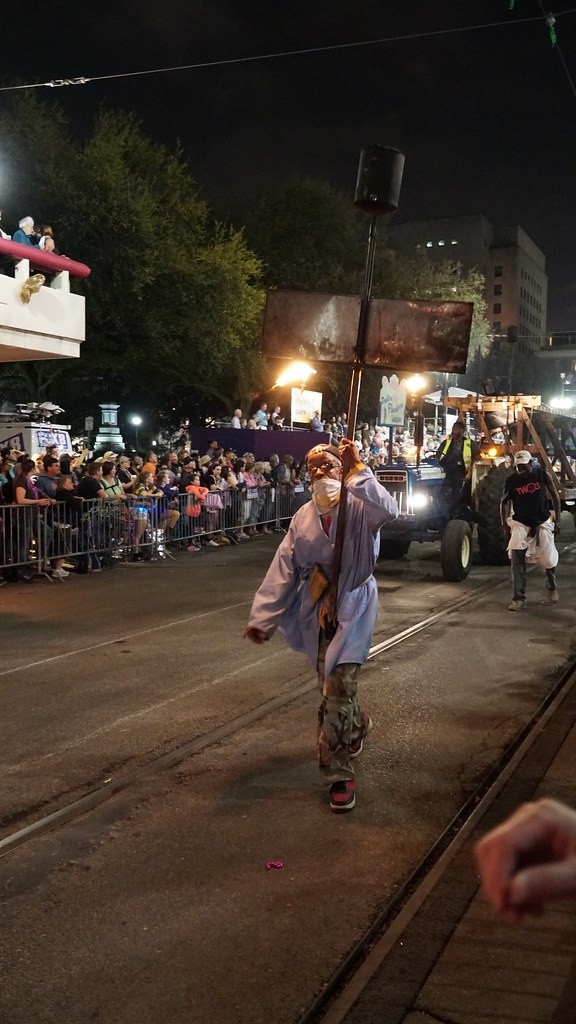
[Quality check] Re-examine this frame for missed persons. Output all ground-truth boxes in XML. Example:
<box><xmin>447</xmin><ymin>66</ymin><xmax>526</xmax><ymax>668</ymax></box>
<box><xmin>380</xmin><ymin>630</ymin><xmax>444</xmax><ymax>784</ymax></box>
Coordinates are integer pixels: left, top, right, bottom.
<box><xmin>435</xmin><ymin>422</ymin><xmax>481</xmax><ymax>521</ymax></box>
<box><xmin>241</xmin><ymin>438</ymin><xmax>398</xmax><ymax>811</ymax></box>
<box><xmin>0</xmin><ymin>403</ymin><xmax>442</xmax><ymax>579</ymax></box>
<box><xmin>0</xmin><ymin>211</ymin><xmax>71</xmax><ymax>287</ymax></box>
<box><xmin>475</xmin><ymin>797</ymin><xmax>576</xmax><ymax>923</ymax></box>
<box><xmin>499</xmin><ymin>449</ymin><xmax>561</xmax><ymax>611</ymax></box>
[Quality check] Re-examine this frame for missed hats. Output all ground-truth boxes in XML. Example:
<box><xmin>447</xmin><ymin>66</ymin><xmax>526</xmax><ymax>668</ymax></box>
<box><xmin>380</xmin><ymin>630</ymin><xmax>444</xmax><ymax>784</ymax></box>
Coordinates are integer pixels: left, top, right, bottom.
<box><xmin>201</xmin><ymin>455</ymin><xmax>212</xmax><ymax>466</ymax></box>
<box><xmin>512</xmin><ymin>450</ymin><xmax>533</xmax><ymax>465</ymax></box>
<box><xmin>305</xmin><ymin>443</ymin><xmax>344</xmax><ymax>464</ymax></box>
<box><xmin>120</xmin><ymin>456</ymin><xmax>131</xmax><ymax>465</ymax></box>
<box><xmin>224</xmin><ymin>447</ymin><xmax>236</xmax><ymax>455</ymax></box>
<box><xmin>103</xmin><ymin>450</ymin><xmax>118</xmax><ymax>459</ymax></box>
<box><xmin>67</xmin><ymin>451</ymin><xmax>80</xmax><ymax>458</ymax></box>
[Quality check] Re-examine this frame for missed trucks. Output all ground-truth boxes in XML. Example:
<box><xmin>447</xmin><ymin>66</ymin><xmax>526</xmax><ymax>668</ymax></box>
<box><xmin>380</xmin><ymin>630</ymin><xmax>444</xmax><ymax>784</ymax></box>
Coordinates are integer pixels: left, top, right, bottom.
<box><xmin>0</xmin><ymin>400</ymin><xmax>73</xmax><ymax>479</ymax></box>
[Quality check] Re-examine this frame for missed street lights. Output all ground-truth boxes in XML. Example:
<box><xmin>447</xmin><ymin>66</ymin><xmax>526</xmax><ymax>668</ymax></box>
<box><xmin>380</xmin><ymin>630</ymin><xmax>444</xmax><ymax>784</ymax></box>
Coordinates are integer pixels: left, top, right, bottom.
<box><xmin>131</xmin><ymin>416</ymin><xmax>142</xmax><ymax>455</ymax></box>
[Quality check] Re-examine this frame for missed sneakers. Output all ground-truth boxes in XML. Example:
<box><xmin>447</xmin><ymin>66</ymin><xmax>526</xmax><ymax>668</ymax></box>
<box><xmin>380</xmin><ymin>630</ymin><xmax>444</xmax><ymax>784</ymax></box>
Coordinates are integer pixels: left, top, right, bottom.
<box><xmin>330</xmin><ymin>777</ymin><xmax>356</xmax><ymax>810</ymax></box>
<box><xmin>52</xmin><ymin>568</ymin><xmax>70</xmax><ymax>577</ymax></box>
<box><xmin>349</xmin><ymin>713</ymin><xmax>372</xmax><ymax>759</ymax></box>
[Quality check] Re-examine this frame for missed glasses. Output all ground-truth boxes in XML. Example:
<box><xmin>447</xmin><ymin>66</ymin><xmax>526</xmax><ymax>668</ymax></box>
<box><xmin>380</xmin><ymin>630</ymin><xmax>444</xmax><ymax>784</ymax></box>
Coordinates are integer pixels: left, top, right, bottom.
<box><xmin>307</xmin><ymin>463</ymin><xmax>342</xmax><ymax>477</ymax></box>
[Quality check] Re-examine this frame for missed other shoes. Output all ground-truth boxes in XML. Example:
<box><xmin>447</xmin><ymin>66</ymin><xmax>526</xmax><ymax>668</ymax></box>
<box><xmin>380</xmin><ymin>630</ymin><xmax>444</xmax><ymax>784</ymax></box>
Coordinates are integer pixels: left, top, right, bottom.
<box><xmin>132</xmin><ymin>552</ymin><xmax>144</xmax><ymax>562</ymax></box>
<box><xmin>547</xmin><ymin>585</ymin><xmax>559</xmax><ymax>602</ymax></box>
<box><xmin>206</xmin><ymin>540</ymin><xmax>220</xmax><ymax>546</ymax></box>
<box><xmin>248</xmin><ymin>530</ymin><xmax>263</xmax><ymax>537</ymax></box>
<box><xmin>238</xmin><ymin>533</ymin><xmax>249</xmax><ymax>538</ymax></box>
<box><xmin>262</xmin><ymin>529</ymin><xmax>272</xmax><ymax>535</ymax></box>
<box><xmin>187</xmin><ymin>546</ymin><xmax>200</xmax><ymax>552</ymax></box>
<box><xmin>508</xmin><ymin>600</ymin><xmax>528</xmax><ymax>610</ymax></box>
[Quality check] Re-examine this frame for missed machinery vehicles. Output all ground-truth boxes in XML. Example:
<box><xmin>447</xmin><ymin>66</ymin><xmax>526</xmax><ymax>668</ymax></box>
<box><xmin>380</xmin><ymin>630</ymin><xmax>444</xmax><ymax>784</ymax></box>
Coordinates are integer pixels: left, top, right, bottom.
<box><xmin>367</xmin><ymin>391</ymin><xmax>539</xmax><ymax>585</ymax></box>
